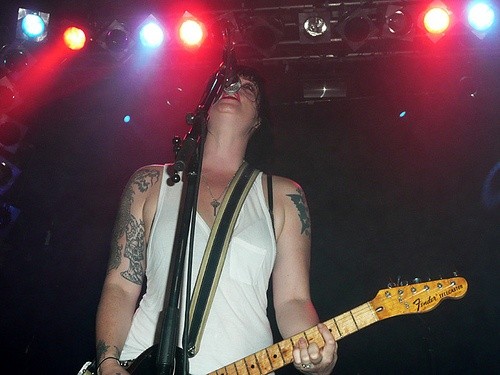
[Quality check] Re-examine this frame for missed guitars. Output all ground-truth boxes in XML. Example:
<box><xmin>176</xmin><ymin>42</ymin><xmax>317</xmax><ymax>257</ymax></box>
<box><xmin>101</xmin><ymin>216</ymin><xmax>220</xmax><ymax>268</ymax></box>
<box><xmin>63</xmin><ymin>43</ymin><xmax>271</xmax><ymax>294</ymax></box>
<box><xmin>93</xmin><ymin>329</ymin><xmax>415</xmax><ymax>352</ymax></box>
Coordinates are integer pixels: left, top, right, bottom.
<box><xmin>79</xmin><ymin>268</ymin><xmax>468</xmax><ymax>375</ymax></box>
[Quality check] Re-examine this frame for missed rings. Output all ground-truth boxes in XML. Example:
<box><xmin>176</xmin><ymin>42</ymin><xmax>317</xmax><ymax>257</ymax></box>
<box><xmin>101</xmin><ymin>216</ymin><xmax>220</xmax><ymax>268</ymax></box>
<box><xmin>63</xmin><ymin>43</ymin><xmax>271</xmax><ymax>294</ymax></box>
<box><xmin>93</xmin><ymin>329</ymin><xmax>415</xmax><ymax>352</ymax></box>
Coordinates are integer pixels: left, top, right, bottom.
<box><xmin>312</xmin><ymin>359</ymin><xmax>322</xmax><ymax>366</ymax></box>
<box><xmin>302</xmin><ymin>362</ymin><xmax>311</xmax><ymax>369</ymax></box>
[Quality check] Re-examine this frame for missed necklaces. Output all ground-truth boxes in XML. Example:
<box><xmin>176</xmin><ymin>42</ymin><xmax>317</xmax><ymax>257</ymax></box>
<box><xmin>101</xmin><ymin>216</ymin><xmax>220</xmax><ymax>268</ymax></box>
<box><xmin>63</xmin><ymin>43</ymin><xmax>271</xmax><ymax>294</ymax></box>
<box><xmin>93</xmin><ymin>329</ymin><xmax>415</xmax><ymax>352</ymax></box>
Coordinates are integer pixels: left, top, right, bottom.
<box><xmin>202</xmin><ymin>162</ymin><xmax>244</xmax><ymax>216</ymax></box>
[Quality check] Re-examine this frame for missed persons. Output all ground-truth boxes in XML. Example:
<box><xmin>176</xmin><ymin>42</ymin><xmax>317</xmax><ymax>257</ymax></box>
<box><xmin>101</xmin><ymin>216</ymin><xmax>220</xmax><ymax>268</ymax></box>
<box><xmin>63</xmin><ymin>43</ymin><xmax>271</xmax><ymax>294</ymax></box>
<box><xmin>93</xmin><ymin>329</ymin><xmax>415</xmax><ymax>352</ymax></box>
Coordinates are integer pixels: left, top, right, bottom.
<box><xmin>96</xmin><ymin>66</ymin><xmax>337</xmax><ymax>375</ymax></box>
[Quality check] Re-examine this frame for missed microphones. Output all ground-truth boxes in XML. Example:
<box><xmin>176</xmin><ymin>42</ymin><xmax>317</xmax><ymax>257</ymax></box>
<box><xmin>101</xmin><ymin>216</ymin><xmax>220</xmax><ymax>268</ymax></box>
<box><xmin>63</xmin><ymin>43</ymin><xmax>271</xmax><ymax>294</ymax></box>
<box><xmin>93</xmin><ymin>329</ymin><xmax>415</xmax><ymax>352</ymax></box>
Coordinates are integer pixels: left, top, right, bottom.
<box><xmin>224</xmin><ymin>28</ymin><xmax>241</xmax><ymax>94</ymax></box>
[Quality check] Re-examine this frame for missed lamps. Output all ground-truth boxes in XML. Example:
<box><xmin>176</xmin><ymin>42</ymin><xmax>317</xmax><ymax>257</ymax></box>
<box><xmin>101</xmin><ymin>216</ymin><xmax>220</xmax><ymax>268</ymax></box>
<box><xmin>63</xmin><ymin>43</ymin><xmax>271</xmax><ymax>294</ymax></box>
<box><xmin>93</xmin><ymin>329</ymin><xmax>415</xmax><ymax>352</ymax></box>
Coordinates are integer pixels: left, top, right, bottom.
<box><xmin>381</xmin><ymin>4</ymin><xmax>416</xmax><ymax>42</ymax></box>
<box><xmin>95</xmin><ymin>18</ymin><xmax>138</xmax><ymax>64</ymax></box>
<box><xmin>298</xmin><ymin>11</ymin><xmax>331</xmax><ymax>44</ymax></box>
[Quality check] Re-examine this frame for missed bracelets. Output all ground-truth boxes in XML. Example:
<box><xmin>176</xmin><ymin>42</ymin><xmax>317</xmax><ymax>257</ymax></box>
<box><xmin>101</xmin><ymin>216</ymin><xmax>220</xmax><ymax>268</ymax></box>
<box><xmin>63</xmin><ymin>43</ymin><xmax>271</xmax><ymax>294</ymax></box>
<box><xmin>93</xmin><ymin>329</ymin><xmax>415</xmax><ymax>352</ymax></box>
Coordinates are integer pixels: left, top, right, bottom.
<box><xmin>95</xmin><ymin>357</ymin><xmax>121</xmax><ymax>370</ymax></box>
